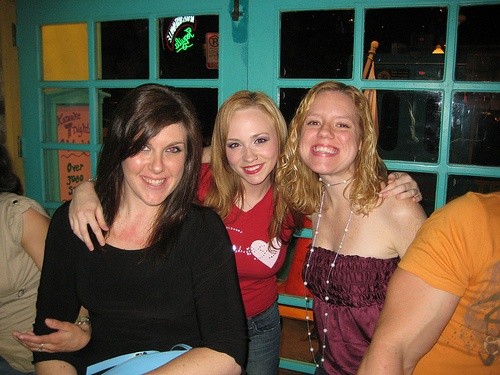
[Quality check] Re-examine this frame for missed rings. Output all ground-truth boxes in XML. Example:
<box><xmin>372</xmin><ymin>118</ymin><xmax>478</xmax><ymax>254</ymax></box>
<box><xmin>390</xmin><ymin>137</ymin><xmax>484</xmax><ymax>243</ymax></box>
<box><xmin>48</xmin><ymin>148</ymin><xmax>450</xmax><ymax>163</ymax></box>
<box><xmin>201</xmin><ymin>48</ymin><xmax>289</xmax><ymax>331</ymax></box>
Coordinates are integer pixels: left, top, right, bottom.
<box><xmin>40</xmin><ymin>343</ymin><xmax>44</xmax><ymax>350</ymax></box>
<box><xmin>393</xmin><ymin>172</ymin><xmax>400</xmax><ymax>179</ymax></box>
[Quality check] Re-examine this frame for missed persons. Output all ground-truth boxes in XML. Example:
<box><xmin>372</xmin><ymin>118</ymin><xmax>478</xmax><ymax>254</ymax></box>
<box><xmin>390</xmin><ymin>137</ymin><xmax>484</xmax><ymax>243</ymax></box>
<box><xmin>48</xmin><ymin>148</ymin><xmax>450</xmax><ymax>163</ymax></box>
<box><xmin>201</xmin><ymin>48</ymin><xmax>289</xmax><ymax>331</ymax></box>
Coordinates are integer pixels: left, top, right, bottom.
<box><xmin>0</xmin><ymin>138</ymin><xmax>92</xmax><ymax>375</ymax></box>
<box><xmin>68</xmin><ymin>90</ymin><xmax>422</xmax><ymax>375</ymax></box>
<box><xmin>358</xmin><ymin>192</ymin><xmax>500</xmax><ymax>375</ymax></box>
<box><xmin>32</xmin><ymin>83</ymin><xmax>250</xmax><ymax>375</ymax></box>
<box><xmin>275</xmin><ymin>80</ymin><xmax>427</xmax><ymax>375</ymax></box>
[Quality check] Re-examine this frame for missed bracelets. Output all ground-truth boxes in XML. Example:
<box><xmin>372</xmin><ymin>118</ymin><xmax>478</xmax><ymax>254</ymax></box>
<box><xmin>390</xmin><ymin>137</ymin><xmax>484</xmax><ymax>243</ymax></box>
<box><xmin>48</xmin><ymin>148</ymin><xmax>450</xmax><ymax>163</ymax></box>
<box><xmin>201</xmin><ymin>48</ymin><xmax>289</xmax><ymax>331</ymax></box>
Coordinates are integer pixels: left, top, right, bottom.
<box><xmin>75</xmin><ymin>316</ymin><xmax>92</xmax><ymax>327</ymax></box>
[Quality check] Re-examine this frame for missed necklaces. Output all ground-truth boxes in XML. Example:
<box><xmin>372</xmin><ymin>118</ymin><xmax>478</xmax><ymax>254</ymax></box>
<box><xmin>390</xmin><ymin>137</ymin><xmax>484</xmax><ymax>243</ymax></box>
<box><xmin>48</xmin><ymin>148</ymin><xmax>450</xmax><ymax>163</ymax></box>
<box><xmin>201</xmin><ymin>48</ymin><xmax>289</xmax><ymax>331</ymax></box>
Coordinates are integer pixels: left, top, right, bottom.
<box><xmin>304</xmin><ymin>178</ymin><xmax>355</xmax><ymax>368</ymax></box>
